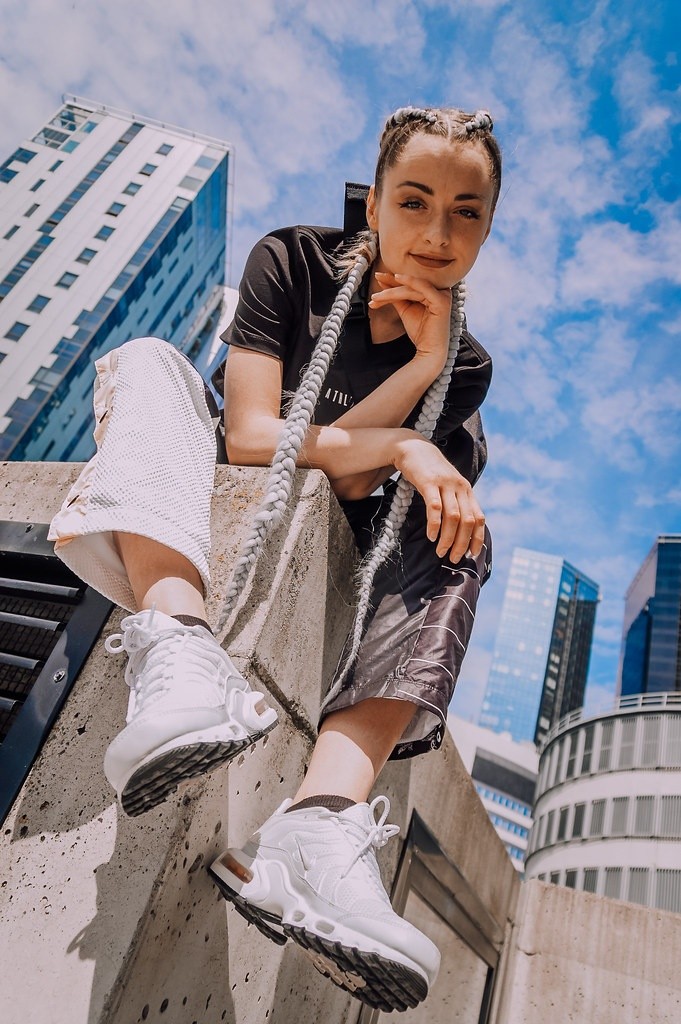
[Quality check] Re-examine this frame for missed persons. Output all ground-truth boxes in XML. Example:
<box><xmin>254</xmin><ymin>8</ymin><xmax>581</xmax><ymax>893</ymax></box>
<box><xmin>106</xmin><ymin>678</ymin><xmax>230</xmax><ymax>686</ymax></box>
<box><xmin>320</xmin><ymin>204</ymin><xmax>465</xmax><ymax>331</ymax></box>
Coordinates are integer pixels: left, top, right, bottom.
<box><xmin>49</xmin><ymin>105</ymin><xmax>505</xmax><ymax>1015</ymax></box>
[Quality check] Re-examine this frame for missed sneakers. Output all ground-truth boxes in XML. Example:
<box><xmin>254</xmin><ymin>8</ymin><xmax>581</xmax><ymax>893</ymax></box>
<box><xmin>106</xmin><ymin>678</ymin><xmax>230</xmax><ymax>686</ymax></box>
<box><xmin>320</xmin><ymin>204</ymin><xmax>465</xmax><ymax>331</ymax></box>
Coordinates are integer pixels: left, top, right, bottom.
<box><xmin>207</xmin><ymin>795</ymin><xmax>440</xmax><ymax>1013</ymax></box>
<box><xmin>102</xmin><ymin>601</ymin><xmax>277</xmax><ymax>818</ymax></box>
